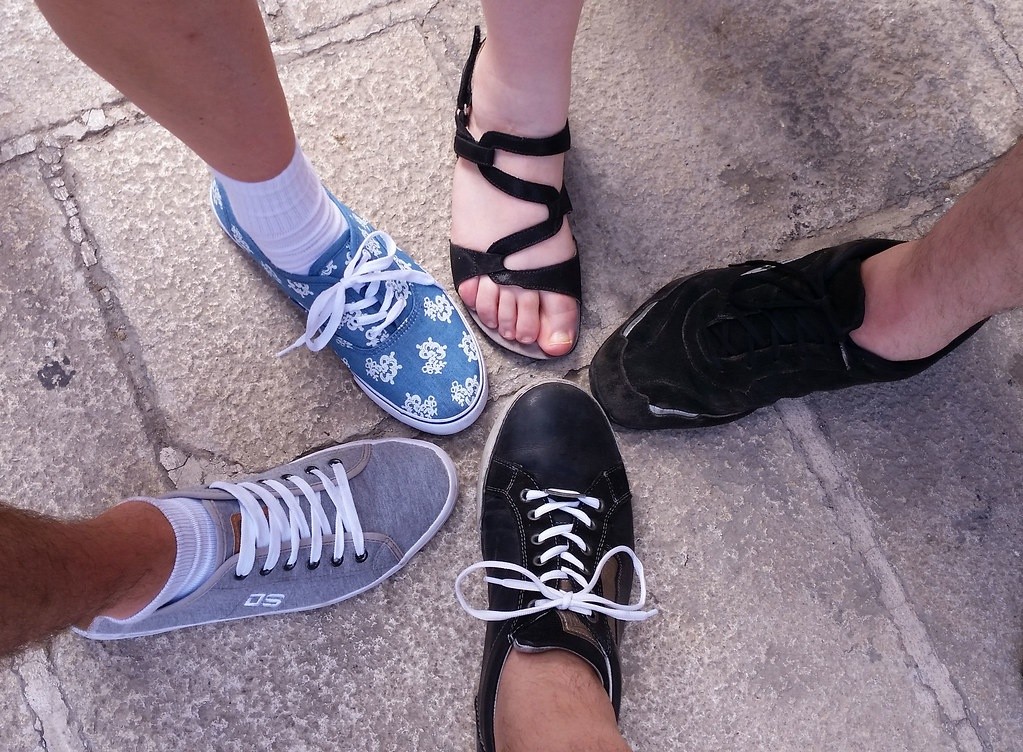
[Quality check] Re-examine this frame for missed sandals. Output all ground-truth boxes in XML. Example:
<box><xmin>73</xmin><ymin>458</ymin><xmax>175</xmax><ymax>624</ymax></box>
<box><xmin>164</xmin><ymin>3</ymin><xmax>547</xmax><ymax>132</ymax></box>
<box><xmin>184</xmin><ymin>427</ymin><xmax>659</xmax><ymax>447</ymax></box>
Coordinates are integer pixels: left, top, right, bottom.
<box><xmin>449</xmin><ymin>25</ymin><xmax>581</xmax><ymax>358</ymax></box>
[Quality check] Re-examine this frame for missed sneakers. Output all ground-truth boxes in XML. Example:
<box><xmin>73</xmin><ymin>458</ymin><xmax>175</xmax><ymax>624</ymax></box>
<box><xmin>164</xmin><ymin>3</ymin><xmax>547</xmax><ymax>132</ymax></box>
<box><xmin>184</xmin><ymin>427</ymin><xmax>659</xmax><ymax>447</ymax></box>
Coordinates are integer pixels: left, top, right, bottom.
<box><xmin>210</xmin><ymin>176</ymin><xmax>488</xmax><ymax>434</ymax></box>
<box><xmin>588</xmin><ymin>239</ymin><xmax>991</xmax><ymax>433</ymax></box>
<box><xmin>69</xmin><ymin>438</ymin><xmax>459</xmax><ymax>640</ymax></box>
<box><xmin>454</xmin><ymin>379</ymin><xmax>660</xmax><ymax>752</ymax></box>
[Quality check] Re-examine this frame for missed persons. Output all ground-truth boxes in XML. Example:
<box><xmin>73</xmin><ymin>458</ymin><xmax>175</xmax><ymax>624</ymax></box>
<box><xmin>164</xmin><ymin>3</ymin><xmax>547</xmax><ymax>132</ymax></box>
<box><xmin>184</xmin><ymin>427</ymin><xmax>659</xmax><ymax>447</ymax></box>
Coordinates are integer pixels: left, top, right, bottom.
<box><xmin>0</xmin><ymin>436</ymin><xmax>459</xmax><ymax>658</ymax></box>
<box><xmin>449</xmin><ymin>0</ymin><xmax>585</xmax><ymax>359</ymax></box>
<box><xmin>589</xmin><ymin>135</ymin><xmax>1022</xmax><ymax>430</ymax></box>
<box><xmin>454</xmin><ymin>379</ymin><xmax>659</xmax><ymax>752</ymax></box>
<box><xmin>34</xmin><ymin>0</ymin><xmax>488</xmax><ymax>434</ymax></box>
